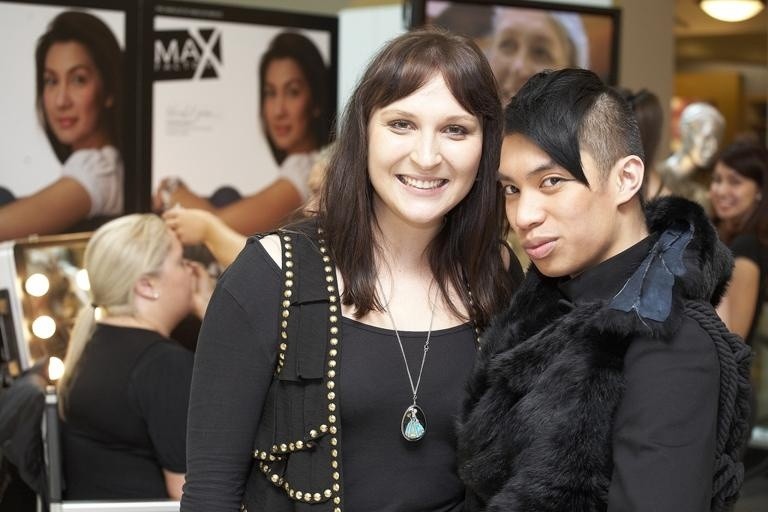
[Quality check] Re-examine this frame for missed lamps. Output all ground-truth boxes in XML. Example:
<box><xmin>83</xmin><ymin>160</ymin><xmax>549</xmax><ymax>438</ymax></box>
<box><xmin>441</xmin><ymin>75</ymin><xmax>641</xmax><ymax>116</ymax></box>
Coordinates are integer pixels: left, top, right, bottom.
<box><xmin>696</xmin><ymin>0</ymin><xmax>766</xmax><ymax>23</ymax></box>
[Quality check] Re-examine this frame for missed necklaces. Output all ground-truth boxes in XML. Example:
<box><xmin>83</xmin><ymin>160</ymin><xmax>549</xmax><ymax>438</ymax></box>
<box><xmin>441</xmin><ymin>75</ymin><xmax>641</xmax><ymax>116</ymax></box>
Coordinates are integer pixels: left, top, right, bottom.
<box><xmin>376</xmin><ymin>276</ymin><xmax>440</xmax><ymax>444</ymax></box>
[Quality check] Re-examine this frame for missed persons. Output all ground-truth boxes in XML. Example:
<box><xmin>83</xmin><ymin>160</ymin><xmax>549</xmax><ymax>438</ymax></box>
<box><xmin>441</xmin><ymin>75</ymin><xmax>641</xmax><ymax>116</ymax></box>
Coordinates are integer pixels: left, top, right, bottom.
<box><xmin>56</xmin><ymin>212</ymin><xmax>215</xmax><ymax>501</ymax></box>
<box><xmin>1</xmin><ymin>11</ymin><xmax>129</xmax><ymax>241</ymax></box>
<box><xmin>178</xmin><ymin>24</ymin><xmax>525</xmax><ymax>511</ymax></box>
<box><xmin>710</xmin><ymin>140</ymin><xmax>768</xmax><ymax>429</ymax></box>
<box><xmin>646</xmin><ymin>99</ymin><xmax>727</xmax><ymax>224</ymax></box>
<box><xmin>151</xmin><ymin>30</ymin><xmax>334</xmax><ymax>240</ymax></box>
<box><xmin>162</xmin><ymin>139</ymin><xmax>339</xmax><ymax>322</ymax></box>
<box><xmin>449</xmin><ymin>66</ymin><xmax>755</xmax><ymax>512</ymax></box>
<box><xmin>485</xmin><ymin>9</ymin><xmax>592</xmax><ymax>112</ymax></box>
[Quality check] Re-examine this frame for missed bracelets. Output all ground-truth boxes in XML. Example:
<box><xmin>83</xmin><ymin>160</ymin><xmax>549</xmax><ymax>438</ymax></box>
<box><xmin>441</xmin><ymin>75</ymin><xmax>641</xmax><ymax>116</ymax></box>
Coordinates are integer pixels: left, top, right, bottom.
<box><xmin>160</xmin><ymin>179</ymin><xmax>183</xmax><ymax>211</ymax></box>
